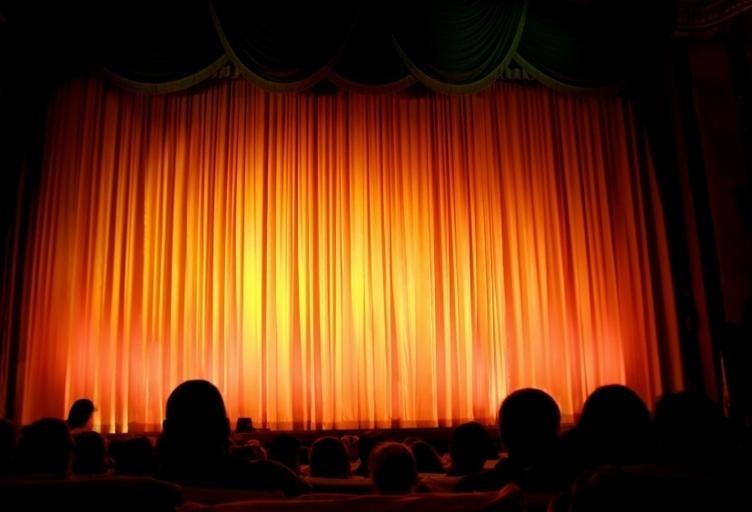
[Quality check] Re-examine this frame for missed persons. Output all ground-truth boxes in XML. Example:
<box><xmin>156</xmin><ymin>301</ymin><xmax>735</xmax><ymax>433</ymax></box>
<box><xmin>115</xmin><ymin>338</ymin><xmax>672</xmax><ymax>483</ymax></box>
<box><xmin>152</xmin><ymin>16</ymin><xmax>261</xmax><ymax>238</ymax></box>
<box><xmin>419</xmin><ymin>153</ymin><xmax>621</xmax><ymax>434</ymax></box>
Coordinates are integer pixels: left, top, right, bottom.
<box><xmin>1</xmin><ymin>378</ymin><xmax>751</xmax><ymax>511</ymax></box>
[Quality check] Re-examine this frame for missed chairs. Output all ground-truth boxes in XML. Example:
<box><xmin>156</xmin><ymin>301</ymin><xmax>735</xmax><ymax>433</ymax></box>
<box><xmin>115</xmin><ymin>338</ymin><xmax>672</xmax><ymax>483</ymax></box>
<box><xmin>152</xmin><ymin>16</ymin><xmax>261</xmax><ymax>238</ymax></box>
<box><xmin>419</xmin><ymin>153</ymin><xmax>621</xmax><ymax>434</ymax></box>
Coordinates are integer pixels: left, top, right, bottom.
<box><xmin>0</xmin><ymin>453</ymin><xmax>752</xmax><ymax>512</ymax></box>
